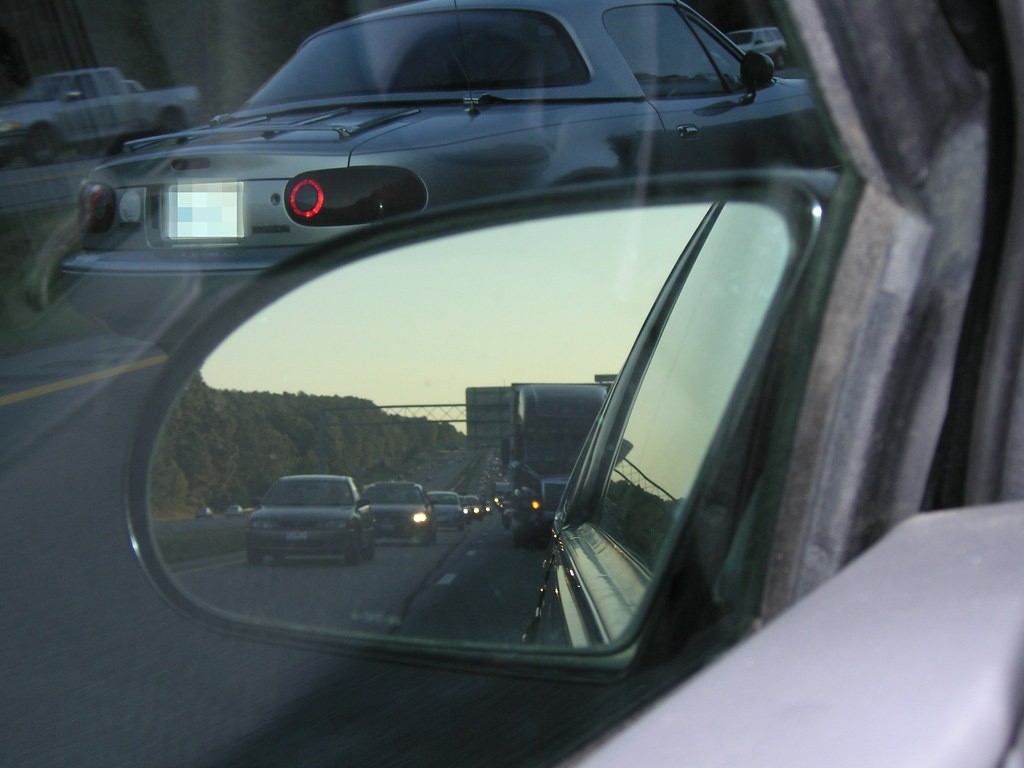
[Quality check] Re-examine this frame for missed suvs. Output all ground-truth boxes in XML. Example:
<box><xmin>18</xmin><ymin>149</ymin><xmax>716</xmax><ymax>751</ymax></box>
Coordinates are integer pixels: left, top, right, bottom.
<box><xmin>727</xmin><ymin>25</ymin><xmax>790</xmax><ymax>70</ymax></box>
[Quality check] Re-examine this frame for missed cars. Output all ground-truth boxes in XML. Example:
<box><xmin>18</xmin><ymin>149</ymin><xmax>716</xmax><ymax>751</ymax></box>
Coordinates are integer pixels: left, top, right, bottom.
<box><xmin>461</xmin><ymin>494</ymin><xmax>486</xmax><ymax>520</ymax></box>
<box><xmin>195</xmin><ymin>507</ymin><xmax>214</xmax><ymax>518</ymax></box>
<box><xmin>358</xmin><ymin>481</ymin><xmax>440</xmax><ymax>546</ymax></box>
<box><xmin>243</xmin><ymin>475</ymin><xmax>383</xmax><ymax>561</ymax></box>
<box><xmin>426</xmin><ymin>491</ymin><xmax>471</xmax><ymax>531</ymax></box>
<box><xmin>225</xmin><ymin>505</ymin><xmax>243</xmax><ymax>517</ymax></box>
<box><xmin>52</xmin><ymin>0</ymin><xmax>832</xmax><ymax>340</ymax></box>
<box><xmin>492</xmin><ymin>481</ymin><xmax>514</xmax><ymax>512</ymax></box>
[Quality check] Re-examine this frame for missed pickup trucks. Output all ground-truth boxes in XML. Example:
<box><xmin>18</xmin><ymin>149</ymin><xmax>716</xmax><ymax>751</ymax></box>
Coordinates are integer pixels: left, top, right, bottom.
<box><xmin>5</xmin><ymin>67</ymin><xmax>205</xmax><ymax>169</ymax></box>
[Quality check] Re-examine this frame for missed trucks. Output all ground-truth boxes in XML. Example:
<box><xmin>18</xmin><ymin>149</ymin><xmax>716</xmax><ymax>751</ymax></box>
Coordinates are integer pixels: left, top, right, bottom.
<box><xmin>509</xmin><ymin>382</ymin><xmax>609</xmax><ymax>548</ymax></box>
